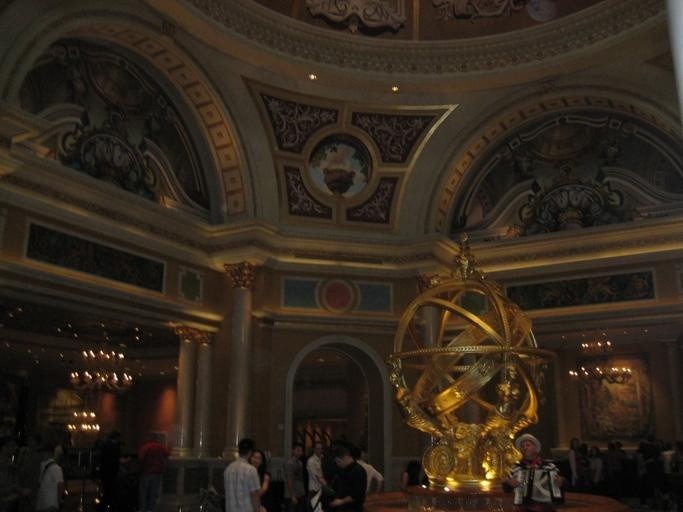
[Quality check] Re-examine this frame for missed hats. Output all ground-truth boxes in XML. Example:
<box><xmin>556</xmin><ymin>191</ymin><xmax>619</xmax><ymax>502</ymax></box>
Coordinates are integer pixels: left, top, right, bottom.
<box><xmin>515</xmin><ymin>434</ymin><xmax>542</xmax><ymax>454</ymax></box>
